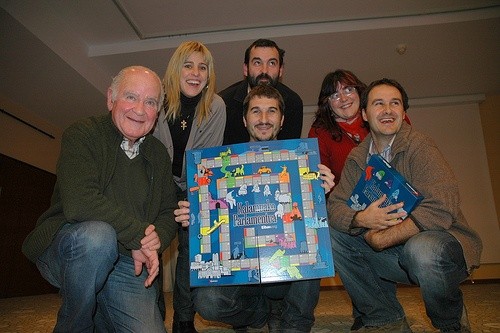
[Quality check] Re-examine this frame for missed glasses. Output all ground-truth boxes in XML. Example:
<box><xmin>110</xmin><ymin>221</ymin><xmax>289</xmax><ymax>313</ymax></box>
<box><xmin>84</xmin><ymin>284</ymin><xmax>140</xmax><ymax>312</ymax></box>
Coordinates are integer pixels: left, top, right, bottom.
<box><xmin>326</xmin><ymin>85</ymin><xmax>356</xmax><ymax>102</ymax></box>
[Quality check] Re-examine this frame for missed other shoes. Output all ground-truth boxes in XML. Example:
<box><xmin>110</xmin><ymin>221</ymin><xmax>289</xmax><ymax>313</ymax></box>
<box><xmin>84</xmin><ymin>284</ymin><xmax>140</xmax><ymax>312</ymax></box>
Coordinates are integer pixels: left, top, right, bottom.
<box><xmin>358</xmin><ymin>320</ymin><xmax>411</xmax><ymax>333</ymax></box>
<box><xmin>436</xmin><ymin>304</ymin><xmax>469</xmax><ymax>333</ymax></box>
<box><xmin>171</xmin><ymin>320</ymin><xmax>198</xmax><ymax>333</ymax></box>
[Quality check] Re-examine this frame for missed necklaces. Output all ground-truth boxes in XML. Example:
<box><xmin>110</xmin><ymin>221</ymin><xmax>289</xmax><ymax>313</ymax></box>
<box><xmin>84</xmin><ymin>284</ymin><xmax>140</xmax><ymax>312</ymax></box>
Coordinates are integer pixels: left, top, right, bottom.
<box><xmin>180</xmin><ymin>114</ymin><xmax>190</xmax><ymax>131</ymax></box>
<box><xmin>190</xmin><ymin>82</ymin><xmax>335</xmax><ymax>333</ymax></box>
<box><xmin>326</xmin><ymin>77</ymin><xmax>483</xmax><ymax>333</ymax></box>
<box><xmin>308</xmin><ymin>68</ymin><xmax>413</xmax><ymax>198</ymax></box>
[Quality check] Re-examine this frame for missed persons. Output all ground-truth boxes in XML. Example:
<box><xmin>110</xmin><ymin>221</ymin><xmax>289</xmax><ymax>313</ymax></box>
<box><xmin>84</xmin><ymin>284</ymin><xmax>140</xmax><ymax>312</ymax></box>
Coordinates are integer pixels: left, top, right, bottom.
<box><xmin>150</xmin><ymin>41</ymin><xmax>226</xmax><ymax>333</ymax></box>
<box><xmin>216</xmin><ymin>38</ymin><xmax>303</xmax><ymax>145</ymax></box>
<box><xmin>18</xmin><ymin>65</ymin><xmax>179</xmax><ymax>333</ymax></box>
